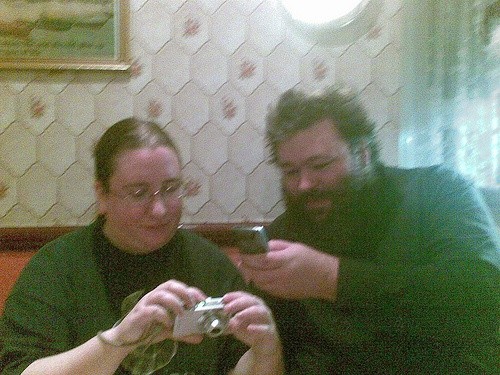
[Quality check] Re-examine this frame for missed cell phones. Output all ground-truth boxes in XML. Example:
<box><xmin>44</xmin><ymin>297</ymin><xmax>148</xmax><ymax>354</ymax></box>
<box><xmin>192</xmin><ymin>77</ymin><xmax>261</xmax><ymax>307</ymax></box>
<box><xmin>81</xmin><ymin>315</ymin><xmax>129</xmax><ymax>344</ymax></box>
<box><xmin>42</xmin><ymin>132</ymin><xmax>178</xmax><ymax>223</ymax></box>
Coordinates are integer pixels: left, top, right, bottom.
<box><xmin>233</xmin><ymin>225</ymin><xmax>269</xmax><ymax>256</ymax></box>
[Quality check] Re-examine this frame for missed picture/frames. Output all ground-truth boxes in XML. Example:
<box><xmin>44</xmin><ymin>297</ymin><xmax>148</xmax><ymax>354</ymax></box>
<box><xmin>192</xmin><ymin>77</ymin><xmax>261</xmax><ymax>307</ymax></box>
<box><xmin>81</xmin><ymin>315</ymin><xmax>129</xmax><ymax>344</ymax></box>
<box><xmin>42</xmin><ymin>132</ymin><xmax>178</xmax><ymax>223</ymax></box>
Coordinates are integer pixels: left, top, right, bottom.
<box><xmin>0</xmin><ymin>0</ymin><xmax>132</xmax><ymax>71</ymax></box>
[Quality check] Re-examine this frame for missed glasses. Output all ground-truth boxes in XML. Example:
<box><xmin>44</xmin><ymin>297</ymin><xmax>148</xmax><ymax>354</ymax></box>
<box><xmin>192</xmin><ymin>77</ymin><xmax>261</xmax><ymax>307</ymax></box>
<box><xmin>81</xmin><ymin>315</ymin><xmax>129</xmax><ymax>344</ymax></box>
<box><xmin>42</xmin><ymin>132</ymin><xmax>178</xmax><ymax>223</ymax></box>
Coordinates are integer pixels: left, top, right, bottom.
<box><xmin>102</xmin><ymin>181</ymin><xmax>186</xmax><ymax>205</ymax></box>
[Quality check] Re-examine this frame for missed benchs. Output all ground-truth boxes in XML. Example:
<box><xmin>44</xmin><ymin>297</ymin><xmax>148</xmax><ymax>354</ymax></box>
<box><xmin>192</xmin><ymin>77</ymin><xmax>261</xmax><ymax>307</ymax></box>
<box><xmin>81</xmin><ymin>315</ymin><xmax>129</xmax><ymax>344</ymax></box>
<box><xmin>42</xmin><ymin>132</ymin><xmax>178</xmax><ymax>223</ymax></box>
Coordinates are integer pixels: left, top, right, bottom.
<box><xmin>0</xmin><ymin>221</ymin><xmax>272</xmax><ymax>318</ymax></box>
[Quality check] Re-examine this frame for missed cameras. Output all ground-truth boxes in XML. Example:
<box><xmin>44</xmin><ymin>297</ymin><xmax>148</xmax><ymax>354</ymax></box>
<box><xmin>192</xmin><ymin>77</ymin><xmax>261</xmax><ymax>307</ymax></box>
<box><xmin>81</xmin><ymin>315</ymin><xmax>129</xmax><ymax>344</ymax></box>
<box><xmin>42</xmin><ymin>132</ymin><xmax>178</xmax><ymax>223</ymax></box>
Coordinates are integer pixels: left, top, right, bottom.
<box><xmin>172</xmin><ymin>296</ymin><xmax>230</xmax><ymax>339</ymax></box>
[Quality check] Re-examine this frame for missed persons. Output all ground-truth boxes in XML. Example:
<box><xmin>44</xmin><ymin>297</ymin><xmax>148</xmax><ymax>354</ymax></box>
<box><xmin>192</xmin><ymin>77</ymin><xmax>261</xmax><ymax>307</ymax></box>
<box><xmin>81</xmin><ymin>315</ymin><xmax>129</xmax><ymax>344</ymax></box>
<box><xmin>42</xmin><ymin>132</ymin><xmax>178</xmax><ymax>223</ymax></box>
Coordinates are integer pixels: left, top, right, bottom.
<box><xmin>0</xmin><ymin>118</ymin><xmax>284</xmax><ymax>375</ymax></box>
<box><xmin>241</xmin><ymin>85</ymin><xmax>500</xmax><ymax>375</ymax></box>
